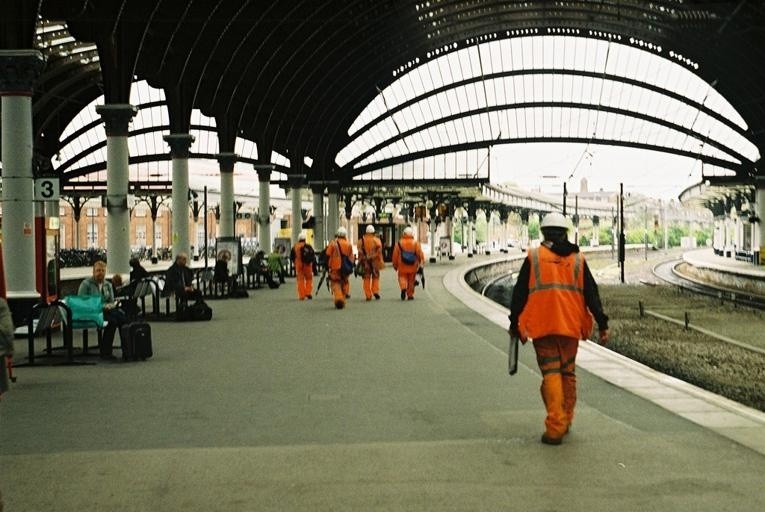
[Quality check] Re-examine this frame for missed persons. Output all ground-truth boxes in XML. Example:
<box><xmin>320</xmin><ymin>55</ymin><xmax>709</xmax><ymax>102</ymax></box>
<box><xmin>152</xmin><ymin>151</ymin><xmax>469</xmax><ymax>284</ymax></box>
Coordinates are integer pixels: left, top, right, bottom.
<box><xmin>290</xmin><ymin>233</ymin><xmax>313</xmax><ymax>300</ymax></box>
<box><xmin>508</xmin><ymin>212</ymin><xmax>610</xmax><ymax>444</ymax></box>
<box><xmin>326</xmin><ymin>227</ymin><xmax>354</xmax><ymax>309</ymax></box>
<box><xmin>78</xmin><ymin>260</ymin><xmax>126</xmax><ymax>360</ymax></box>
<box><xmin>166</xmin><ymin>253</ymin><xmax>202</xmax><ymax>303</ymax></box>
<box><xmin>267</xmin><ymin>248</ymin><xmax>285</xmax><ymax>283</ymax></box>
<box><xmin>129</xmin><ymin>258</ymin><xmax>148</xmax><ymax>314</ymax></box>
<box><xmin>54</xmin><ymin>247</ymin><xmax>107</xmax><ymax>268</ymax></box>
<box><xmin>357</xmin><ymin>225</ymin><xmax>385</xmax><ymax>301</ymax></box>
<box><xmin>248</xmin><ymin>251</ymin><xmax>279</xmax><ymax>288</ymax></box>
<box><xmin>392</xmin><ymin>226</ymin><xmax>424</xmax><ymax>300</ymax></box>
<box><xmin>214</xmin><ymin>255</ymin><xmax>229</xmax><ymax>281</ymax></box>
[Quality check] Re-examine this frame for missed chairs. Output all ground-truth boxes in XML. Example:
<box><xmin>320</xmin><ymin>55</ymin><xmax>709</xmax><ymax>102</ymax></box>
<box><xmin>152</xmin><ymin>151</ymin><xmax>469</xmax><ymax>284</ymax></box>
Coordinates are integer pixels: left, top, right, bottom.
<box><xmin>238</xmin><ymin>252</ymin><xmax>324</xmax><ymax>290</ymax></box>
<box><xmin>14</xmin><ymin>300</ymin><xmax>137</xmax><ymax>366</ymax></box>
<box><xmin>124</xmin><ymin>266</ymin><xmax>231</xmax><ymax>321</ymax></box>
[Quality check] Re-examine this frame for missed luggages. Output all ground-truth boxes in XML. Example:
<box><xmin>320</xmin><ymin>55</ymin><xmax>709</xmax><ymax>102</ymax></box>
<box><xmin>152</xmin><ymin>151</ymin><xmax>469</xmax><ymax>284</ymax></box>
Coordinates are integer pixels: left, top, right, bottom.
<box><xmin>121</xmin><ymin>322</ymin><xmax>152</xmax><ymax>360</ymax></box>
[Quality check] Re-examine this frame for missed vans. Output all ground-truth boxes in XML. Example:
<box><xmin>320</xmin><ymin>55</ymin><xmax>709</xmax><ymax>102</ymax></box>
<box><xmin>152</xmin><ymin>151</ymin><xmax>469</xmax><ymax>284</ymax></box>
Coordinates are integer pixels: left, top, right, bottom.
<box><xmin>241</xmin><ymin>237</ymin><xmax>257</xmax><ymax>247</ymax></box>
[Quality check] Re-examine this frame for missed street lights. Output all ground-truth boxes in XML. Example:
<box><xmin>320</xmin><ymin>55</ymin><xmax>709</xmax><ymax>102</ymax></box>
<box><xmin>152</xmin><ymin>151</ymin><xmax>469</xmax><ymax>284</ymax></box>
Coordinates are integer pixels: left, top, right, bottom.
<box><xmin>455</xmin><ymin>205</ymin><xmax>464</xmax><ymax>254</ymax></box>
<box><xmin>426</xmin><ymin>199</ymin><xmax>437</xmax><ymax>264</ymax></box>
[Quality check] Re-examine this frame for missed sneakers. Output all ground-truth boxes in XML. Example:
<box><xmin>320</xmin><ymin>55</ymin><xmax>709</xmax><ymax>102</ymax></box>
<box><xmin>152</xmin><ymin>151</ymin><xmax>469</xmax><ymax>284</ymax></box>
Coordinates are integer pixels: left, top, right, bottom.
<box><xmin>541</xmin><ymin>432</ymin><xmax>561</xmax><ymax>445</ymax></box>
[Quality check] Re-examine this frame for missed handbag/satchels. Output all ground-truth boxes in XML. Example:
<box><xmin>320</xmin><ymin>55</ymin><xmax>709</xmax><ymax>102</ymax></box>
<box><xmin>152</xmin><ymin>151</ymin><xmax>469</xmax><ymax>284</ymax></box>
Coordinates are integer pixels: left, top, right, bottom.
<box><xmin>65</xmin><ymin>296</ymin><xmax>103</xmax><ymax>328</ymax></box>
<box><xmin>401</xmin><ymin>251</ymin><xmax>416</xmax><ymax>264</ymax></box>
<box><xmin>341</xmin><ymin>255</ymin><xmax>353</xmax><ymax>277</ymax></box>
<box><xmin>356</xmin><ymin>259</ymin><xmax>364</xmax><ymax>274</ymax></box>
<box><xmin>301</xmin><ymin>244</ymin><xmax>314</xmax><ymax>263</ymax></box>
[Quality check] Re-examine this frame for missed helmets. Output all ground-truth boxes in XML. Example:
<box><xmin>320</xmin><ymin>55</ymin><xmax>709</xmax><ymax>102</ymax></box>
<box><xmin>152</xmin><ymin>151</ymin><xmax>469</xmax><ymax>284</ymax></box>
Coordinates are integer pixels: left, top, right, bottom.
<box><xmin>404</xmin><ymin>227</ymin><xmax>412</xmax><ymax>236</ymax></box>
<box><xmin>366</xmin><ymin>225</ymin><xmax>374</xmax><ymax>233</ymax></box>
<box><xmin>337</xmin><ymin>227</ymin><xmax>346</xmax><ymax>237</ymax></box>
<box><xmin>541</xmin><ymin>213</ymin><xmax>569</xmax><ymax>230</ymax></box>
<box><xmin>298</xmin><ymin>232</ymin><xmax>306</xmax><ymax>241</ymax></box>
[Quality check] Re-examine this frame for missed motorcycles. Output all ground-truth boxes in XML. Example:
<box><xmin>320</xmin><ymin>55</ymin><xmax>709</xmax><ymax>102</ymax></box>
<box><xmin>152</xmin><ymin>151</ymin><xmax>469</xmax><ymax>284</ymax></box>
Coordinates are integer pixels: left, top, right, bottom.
<box><xmin>198</xmin><ymin>241</ymin><xmax>259</xmax><ymax>259</ymax></box>
<box><xmin>132</xmin><ymin>246</ymin><xmax>171</xmax><ymax>262</ymax></box>
<box><xmin>59</xmin><ymin>246</ymin><xmax>106</xmax><ymax>268</ymax></box>
